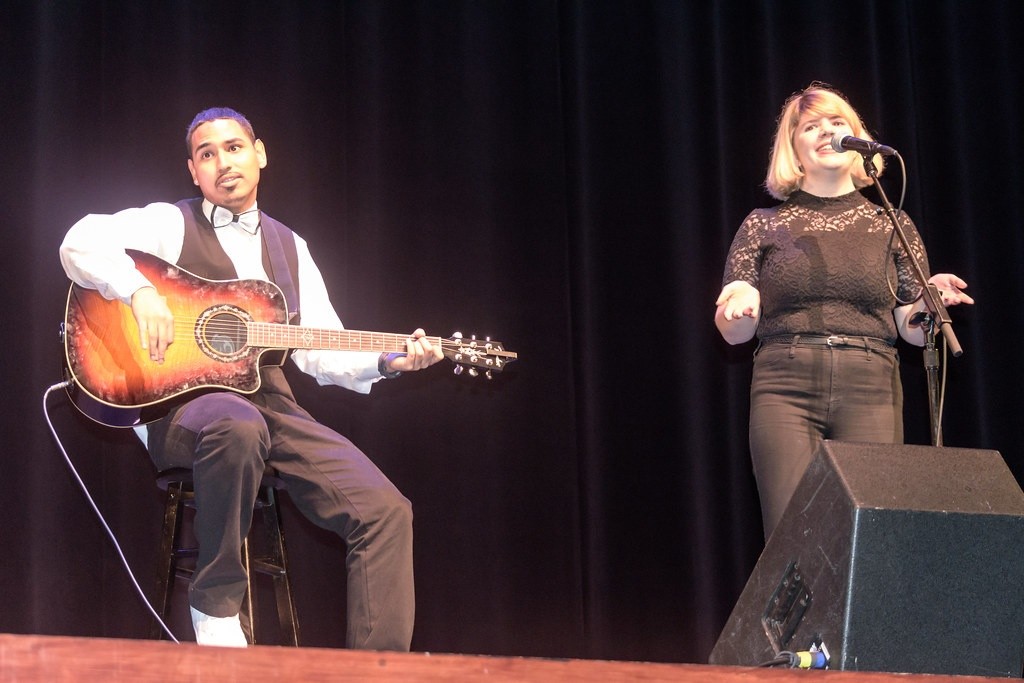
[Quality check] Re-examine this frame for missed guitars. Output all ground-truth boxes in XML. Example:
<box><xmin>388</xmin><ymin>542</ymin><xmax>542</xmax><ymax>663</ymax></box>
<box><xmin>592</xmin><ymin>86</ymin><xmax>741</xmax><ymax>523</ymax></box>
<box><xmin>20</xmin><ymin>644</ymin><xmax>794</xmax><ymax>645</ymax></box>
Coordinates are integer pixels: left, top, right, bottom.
<box><xmin>58</xmin><ymin>246</ymin><xmax>519</xmax><ymax>430</ymax></box>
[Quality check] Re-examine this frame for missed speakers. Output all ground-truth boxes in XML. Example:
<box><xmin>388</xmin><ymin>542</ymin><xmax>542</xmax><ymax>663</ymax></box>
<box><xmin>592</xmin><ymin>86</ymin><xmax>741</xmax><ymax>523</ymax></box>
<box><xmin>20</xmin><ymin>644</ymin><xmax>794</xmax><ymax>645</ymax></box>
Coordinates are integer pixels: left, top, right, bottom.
<box><xmin>708</xmin><ymin>438</ymin><xmax>1024</xmax><ymax>678</ymax></box>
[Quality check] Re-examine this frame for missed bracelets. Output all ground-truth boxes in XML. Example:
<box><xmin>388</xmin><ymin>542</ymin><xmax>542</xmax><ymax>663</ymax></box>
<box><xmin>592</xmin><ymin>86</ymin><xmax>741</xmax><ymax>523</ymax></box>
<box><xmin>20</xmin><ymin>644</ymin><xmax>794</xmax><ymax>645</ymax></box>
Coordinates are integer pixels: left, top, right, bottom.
<box><xmin>378</xmin><ymin>351</ymin><xmax>406</xmax><ymax>379</ymax></box>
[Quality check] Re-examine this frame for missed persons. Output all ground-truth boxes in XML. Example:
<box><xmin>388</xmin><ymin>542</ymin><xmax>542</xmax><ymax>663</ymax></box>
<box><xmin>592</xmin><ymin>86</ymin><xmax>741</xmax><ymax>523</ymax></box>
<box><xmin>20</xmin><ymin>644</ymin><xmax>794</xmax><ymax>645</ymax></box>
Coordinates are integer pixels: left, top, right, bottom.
<box><xmin>714</xmin><ymin>84</ymin><xmax>976</xmax><ymax>541</ymax></box>
<box><xmin>63</xmin><ymin>108</ymin><xmax>444</xmax><ymax>653</ymax></box>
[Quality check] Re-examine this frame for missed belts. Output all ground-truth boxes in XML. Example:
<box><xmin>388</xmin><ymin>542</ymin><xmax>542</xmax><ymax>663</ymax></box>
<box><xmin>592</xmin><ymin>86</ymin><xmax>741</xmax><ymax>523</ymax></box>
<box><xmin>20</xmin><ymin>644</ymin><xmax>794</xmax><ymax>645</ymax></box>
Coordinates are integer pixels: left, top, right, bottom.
<box><xmin>761</xmin><ymin>334</ymin><xmax>898</xmax><ymax>355</ymax></box>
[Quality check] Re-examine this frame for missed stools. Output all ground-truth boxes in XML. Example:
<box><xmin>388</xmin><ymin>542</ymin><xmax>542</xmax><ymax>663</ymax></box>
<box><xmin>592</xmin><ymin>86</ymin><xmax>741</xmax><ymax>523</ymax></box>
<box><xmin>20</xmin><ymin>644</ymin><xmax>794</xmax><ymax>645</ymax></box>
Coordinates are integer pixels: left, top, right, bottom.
<box><xmin>149</xmin><ymin>466</ymin><xmax>303</xmax><ymax>647</ymax></box>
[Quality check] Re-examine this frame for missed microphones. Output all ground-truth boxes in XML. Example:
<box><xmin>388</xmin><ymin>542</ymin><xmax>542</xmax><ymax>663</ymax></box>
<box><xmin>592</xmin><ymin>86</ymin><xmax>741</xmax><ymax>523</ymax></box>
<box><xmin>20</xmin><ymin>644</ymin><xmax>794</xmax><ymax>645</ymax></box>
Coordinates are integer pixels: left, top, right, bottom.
<box><xmin>831</xmin><ymin>133</ymin><xmax>898</xmax><ymax>156</ymax></box>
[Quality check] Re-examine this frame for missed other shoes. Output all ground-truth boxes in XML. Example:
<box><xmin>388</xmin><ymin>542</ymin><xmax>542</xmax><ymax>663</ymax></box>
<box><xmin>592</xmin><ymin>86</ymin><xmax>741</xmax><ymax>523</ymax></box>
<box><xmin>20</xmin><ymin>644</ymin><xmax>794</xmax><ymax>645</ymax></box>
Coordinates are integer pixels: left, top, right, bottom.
<box><xmin>190</xmin><ymin>605</ymin><xmax>248</xmax><ymax>647</ymax></box>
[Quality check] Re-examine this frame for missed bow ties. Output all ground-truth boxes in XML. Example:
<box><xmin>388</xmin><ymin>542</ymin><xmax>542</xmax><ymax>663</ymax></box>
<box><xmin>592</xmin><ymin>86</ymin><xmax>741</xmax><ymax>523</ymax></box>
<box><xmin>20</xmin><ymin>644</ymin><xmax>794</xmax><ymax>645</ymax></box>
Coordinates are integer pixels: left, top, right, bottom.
<box><xmin>211</xmin><ymin>205</ymin><xmax>262</xmax><ymax>235</ymax></box>
